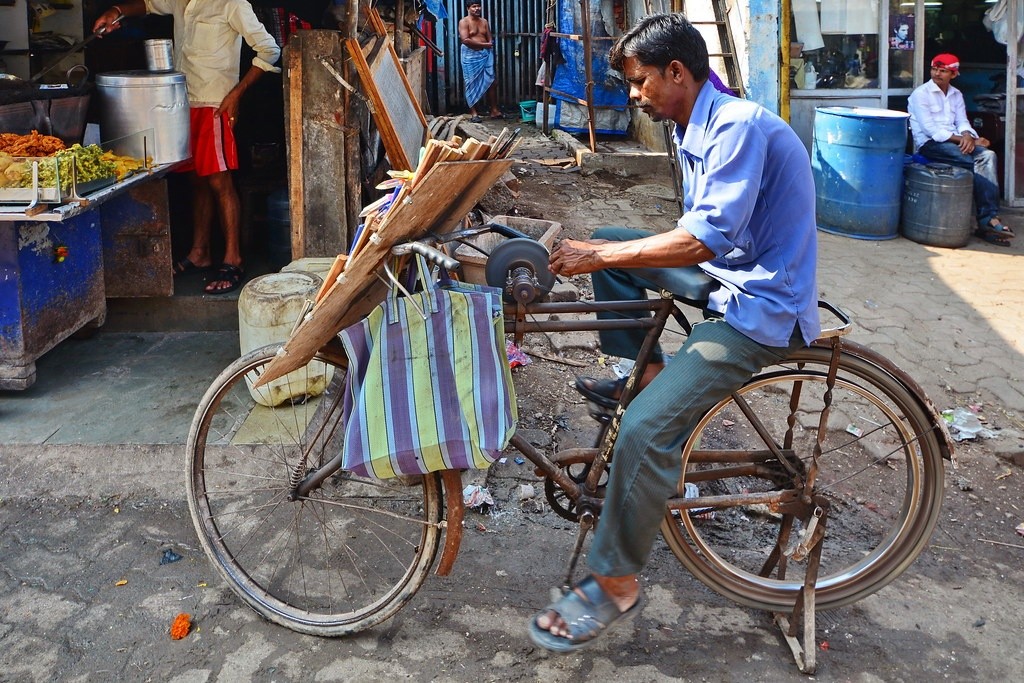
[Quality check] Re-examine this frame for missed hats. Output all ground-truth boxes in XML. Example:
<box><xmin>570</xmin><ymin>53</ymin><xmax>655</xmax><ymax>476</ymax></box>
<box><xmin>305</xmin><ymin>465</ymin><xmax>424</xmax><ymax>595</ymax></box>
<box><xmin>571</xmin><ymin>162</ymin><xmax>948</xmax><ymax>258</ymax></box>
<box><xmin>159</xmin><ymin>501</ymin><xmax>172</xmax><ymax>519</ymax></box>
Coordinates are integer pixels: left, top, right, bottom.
<box><xmin>930</xmin><ymin>53</ymin><xmax>960</xmax><ymax>76</ymax></box>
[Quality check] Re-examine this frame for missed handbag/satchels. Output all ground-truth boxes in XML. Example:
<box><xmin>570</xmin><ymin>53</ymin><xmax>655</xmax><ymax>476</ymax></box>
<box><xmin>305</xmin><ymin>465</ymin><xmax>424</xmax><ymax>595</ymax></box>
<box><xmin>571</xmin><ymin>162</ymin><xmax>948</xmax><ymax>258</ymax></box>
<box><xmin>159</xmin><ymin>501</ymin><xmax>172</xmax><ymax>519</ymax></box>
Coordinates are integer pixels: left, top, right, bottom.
<box><xmin>336</xmin><ymin>255</ymin><xmax>517</xmax><ymax>478</ymax></box>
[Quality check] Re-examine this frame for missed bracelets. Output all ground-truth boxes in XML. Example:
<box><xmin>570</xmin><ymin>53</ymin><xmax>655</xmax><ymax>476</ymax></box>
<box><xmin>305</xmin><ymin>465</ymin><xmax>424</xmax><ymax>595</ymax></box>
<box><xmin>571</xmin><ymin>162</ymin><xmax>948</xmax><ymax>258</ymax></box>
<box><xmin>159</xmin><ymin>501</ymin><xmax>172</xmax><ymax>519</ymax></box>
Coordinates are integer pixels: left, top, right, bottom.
<box><xmin>113</xmin><ymin>4</ymin><xmax>122</xmax><ymax>14</ymax></box>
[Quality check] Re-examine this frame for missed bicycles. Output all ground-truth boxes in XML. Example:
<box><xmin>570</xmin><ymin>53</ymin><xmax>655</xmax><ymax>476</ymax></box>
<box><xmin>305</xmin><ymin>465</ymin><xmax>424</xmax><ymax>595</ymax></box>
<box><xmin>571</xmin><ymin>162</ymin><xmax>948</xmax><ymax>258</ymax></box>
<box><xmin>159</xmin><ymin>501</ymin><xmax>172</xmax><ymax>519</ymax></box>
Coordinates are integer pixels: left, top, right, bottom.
<box><xmin>184</xmin><ymin>223</ymin><xmax>955</xmax><ymax>674</ymax></box>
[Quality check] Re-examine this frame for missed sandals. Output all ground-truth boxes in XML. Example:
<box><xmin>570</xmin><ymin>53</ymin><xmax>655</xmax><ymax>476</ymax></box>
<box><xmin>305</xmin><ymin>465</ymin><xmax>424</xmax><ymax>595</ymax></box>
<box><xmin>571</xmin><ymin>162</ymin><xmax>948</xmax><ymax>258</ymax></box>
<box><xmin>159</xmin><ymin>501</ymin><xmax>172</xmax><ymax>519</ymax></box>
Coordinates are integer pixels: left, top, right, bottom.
<box><xmin>974</xmin><ymin>228</ymin><xmax>1011</xmax><ymax>247</ymax></box>
<box><xmin>976</xmin><ymin>215</ymin><xmax>1015</xmax><ymax>237</ymax></box>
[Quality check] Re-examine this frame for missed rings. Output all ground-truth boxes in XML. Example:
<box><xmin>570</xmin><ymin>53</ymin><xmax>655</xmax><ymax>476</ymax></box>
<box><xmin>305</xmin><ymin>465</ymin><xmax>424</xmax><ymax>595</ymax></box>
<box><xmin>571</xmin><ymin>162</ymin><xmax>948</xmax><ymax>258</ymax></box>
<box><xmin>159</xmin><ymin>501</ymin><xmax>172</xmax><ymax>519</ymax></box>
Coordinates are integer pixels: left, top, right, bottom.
<box><xmin>230</xmin><ymin>117</ymin><xmax>235</xmax><ymax>120</ymax></box>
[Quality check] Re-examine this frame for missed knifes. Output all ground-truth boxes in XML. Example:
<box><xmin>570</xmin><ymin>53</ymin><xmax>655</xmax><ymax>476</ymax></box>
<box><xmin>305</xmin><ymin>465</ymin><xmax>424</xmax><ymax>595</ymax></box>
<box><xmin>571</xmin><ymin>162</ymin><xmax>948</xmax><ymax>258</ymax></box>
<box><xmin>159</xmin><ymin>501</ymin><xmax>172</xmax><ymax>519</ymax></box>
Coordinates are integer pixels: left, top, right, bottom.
<box><xmin>27</xmin><ymin>13</ymin><xmax>124</xmax><ymax>82</ymax></box>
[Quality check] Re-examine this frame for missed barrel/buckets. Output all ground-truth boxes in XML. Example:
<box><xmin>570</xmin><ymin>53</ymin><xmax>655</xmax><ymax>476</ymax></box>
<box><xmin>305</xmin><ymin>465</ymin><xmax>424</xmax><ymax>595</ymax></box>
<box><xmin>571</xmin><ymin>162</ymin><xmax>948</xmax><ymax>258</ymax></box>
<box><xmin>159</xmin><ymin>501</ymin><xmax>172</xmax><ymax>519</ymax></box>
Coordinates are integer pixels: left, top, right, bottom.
<box><xmin>238</xmin><ymin>270</ymin><xmax>334</xmax><ymax>407</ymax></box>
<box><xmin>95</xmin><ymin>70</ymin><xmax>193</xmax><ymax>164</ymax></box>
<box><xmin>281</xmin><ymin>257</ymin><xmax>336</xmax><ymax>279</ymax></box>
<box><xmin>904</xmin><ymin>161</ymin><xmax>974</xmax><ymax>248</ymax></box>
<box><xmin>520</xmin><ymin>101</ymin><xmax>536</xmax><ymax>121</ymax></box>
<box><xmin>809</xmin><ymin>106</ymin><xmax>910</xmax><ymax>242</ymax></box>
<box><xmin>0</xmin><ymin>66</ymin><xmax>91</xmax><ymax>146</ymax></box>
<box><xmin>145</xmin><ymin>39</ymin><xmax>174</xmax><ymax>72</ymax></box>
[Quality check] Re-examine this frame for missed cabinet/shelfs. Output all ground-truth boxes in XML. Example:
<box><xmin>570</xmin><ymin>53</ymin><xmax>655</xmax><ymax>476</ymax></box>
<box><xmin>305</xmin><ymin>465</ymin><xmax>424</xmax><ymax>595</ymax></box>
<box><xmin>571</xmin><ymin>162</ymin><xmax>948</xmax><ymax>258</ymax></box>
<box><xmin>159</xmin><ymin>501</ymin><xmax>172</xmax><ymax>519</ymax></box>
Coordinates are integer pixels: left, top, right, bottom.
<box><xmin>0</xmin><ymin>0</ymin><xmax>89</xmax><ymax>80</ymax></box>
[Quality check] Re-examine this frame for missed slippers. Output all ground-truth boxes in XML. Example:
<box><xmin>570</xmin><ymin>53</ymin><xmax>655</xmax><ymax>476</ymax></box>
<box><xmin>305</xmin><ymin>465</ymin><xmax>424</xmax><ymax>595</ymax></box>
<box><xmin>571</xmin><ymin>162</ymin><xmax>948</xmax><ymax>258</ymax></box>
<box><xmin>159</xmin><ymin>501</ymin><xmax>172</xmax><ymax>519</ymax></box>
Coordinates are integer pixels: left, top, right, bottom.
<box><xmin>528</xmin><ymin>574</ymin><xmax>643</xmax><ymax>652</ymax></box>
<box><xmin>575</xmin><ymin>374</ymin><xmax>629</xmax><ymax>405</ymax></box>
<box><xmin>471</xmin><ymin>116</ymin><xmax>482</xmax><ymax>122</ymax></box>
<box><xmin>172</xmin><ymin>257</ymin><xmax>211</xmax><ymax>278</ymax></box>
<box><xmin>490</xmin><ymin>111</ymin><xmax>513</xmax><ymax>119</ymax></box>
<box><xmin>204</xmin><ymin>263</ymin><xmax>246</xmax><ymax>293</ymax></box>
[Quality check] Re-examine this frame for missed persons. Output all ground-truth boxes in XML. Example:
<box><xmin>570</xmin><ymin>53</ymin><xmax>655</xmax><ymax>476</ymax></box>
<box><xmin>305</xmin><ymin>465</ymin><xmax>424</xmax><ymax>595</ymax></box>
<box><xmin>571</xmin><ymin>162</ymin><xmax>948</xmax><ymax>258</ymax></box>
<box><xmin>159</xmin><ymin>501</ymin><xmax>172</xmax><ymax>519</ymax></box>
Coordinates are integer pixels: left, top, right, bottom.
<box><xmin>527</xmin><ymin>13</ymin><xmax>822</xmax><ymax>653</ymax></box>
<box><xmin>908</xmin><ymin>54</ymin><xmax>1016</xmax><ymax>246</ymax></box>
<box><xmin>92</xmin><ymin>0</ymin><xmax>281</xmax><ymax>294</ymax></box>
<box><xmin>889</xmin><ymin>19</ymin><xmax>914</xmax><ymax>50</ymax></box>
<box><xmin>457</xmin><ymin>0</ymin><xmax>507</xmax><ymax>123</ymax></box>
